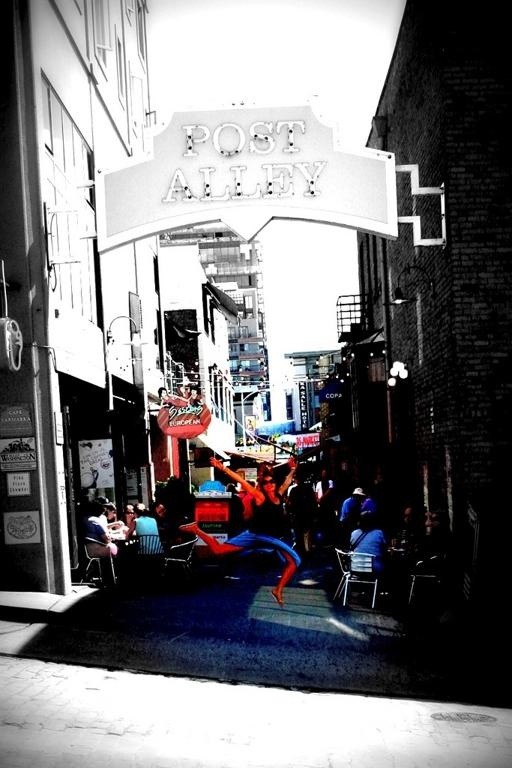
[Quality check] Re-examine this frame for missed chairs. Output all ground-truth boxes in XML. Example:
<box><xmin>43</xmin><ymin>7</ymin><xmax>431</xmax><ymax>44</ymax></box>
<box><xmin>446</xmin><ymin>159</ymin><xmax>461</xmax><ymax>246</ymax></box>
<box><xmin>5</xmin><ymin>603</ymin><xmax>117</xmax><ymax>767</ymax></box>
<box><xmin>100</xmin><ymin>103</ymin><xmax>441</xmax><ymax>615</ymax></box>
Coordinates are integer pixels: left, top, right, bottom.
<box><xmin>333</xmin><ymin>548</ymin><xmax>378</xmax><ymax>609</ymax></box>
<box><xmin>71</xmin><ymin>536</ymin><xmax>199</xmax><ymax>585</ymax></box>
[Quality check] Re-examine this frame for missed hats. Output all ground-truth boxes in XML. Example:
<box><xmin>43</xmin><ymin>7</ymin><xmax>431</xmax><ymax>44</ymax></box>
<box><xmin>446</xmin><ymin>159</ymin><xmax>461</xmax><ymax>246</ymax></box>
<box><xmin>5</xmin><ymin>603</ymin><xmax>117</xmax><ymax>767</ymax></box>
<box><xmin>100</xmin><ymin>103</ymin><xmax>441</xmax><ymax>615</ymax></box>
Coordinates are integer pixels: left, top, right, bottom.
<box><xmin>353</xmin><ymin>488</ymin><xmax>366</xmax><ymax>495</ymax></box>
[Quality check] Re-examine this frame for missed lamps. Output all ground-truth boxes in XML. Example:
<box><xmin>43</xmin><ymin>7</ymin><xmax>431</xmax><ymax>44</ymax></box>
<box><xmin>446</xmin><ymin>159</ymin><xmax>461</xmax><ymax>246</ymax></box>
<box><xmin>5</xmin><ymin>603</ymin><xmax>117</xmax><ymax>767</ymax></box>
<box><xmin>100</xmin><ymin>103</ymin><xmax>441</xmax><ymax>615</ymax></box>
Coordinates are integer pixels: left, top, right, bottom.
<box><xmin>388</xmin><ymin>264</ymin><xmax>433</xmax><ymax>304</ymax></box>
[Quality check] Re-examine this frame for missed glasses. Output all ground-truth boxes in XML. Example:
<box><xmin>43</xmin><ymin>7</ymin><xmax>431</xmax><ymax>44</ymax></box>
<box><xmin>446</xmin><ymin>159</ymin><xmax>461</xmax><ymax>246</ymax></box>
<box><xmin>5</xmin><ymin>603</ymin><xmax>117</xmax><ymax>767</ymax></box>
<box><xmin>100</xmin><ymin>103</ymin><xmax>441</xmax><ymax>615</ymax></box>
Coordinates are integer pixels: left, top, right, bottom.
<box><xmin>262</xmin><ymin>478</ymin><xmax>277</xmax><ymax>485</ymax></box>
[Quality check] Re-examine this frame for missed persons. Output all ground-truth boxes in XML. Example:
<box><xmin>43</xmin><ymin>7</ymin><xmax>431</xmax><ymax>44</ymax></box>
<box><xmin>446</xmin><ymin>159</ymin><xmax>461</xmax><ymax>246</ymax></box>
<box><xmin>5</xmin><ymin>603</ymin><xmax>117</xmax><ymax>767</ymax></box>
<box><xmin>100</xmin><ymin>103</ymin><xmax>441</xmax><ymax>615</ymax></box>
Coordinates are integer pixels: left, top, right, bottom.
<box><xmin>158</xmin><ymin>387</ymin><xmax>186</xmax><ymax>407</ymax></box>
<box><xmin>187</xmin><ymin>388</ymin><xmax>204</xmax><ymax>415</ymax></box>
<box><xmin>84</xmin><ymin>455</ymin><xmax>425</xmax><ymax>610</ymax></box>
